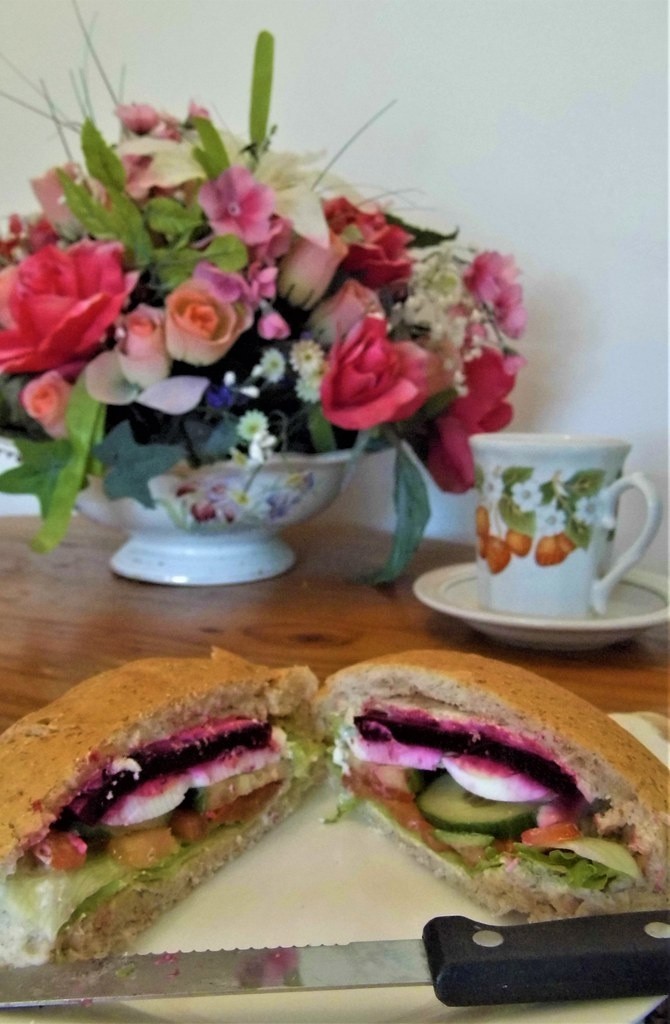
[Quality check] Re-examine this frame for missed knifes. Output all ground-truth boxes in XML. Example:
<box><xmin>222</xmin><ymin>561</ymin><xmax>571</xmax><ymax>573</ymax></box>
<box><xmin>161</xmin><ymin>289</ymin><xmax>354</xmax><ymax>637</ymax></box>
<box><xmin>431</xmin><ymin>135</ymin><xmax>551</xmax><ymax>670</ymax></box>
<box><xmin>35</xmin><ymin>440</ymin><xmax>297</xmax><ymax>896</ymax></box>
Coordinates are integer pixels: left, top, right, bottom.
<box><xmin>0</xmin><ymin>910</ymin><xmax>670</xmax><ymax>1009</ymax></box>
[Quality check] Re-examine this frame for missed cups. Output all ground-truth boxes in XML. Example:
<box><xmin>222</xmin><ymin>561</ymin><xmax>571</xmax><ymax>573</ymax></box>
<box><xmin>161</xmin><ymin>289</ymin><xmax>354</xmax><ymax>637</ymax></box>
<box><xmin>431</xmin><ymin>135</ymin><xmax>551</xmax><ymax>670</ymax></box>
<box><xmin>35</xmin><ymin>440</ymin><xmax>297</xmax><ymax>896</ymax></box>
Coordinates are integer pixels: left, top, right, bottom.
<box><xmin>468</xmin><ymin>431</ymin><xmax>662</xmax><ymax>618</ymax></box>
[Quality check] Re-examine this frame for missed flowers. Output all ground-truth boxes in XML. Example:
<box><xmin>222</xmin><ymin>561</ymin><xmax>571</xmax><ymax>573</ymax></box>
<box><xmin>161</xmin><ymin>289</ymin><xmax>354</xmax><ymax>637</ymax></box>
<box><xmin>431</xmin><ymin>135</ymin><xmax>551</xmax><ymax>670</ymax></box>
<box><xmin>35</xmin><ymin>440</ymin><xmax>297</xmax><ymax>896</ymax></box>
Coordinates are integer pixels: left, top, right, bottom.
<box><xmin>0</xmin><ymin>1</ymin><xmax>527</xmax><ymax>586</ymax></box>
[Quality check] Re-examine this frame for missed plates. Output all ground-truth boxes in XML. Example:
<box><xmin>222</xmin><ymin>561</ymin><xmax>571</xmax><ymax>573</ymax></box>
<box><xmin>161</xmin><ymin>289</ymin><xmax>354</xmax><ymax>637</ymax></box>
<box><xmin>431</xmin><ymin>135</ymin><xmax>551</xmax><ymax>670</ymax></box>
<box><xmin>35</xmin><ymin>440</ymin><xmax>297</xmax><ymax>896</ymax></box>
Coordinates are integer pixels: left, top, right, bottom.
<box><xmin>413</xmin><ymin>561</ymin><xmax>670</xmax><ymax>651</ymax></box>
<box><xmin>0</xmin><ymin>711</ymin><xmax>670</xmax><ymax>1024</ymax></box>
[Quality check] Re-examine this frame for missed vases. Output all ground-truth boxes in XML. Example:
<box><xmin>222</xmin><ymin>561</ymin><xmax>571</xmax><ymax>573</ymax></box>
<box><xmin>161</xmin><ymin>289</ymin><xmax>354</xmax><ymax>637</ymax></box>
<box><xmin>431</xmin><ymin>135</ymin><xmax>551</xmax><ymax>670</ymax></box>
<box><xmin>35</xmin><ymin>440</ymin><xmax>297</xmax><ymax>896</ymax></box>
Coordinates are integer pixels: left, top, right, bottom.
<box><xmin>72</xmin><ymin>448</ymin><xmax>373</xmax><ymax>584</ymax></box>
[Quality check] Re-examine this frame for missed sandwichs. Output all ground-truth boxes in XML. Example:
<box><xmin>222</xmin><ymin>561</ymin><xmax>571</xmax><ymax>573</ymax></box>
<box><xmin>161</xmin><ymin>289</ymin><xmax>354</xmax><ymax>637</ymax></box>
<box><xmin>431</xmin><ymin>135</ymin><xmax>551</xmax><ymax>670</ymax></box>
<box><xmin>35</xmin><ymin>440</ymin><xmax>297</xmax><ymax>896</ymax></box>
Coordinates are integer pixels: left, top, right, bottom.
<box><xmin>307</xmin><ymin>650</ymin><xmax>670</xmax><ymax>922</ymax></box>
<box><xmin>0</xmin><ymin>646</ymin><xmax>326</xmax><ymax>966</ymax></box>
